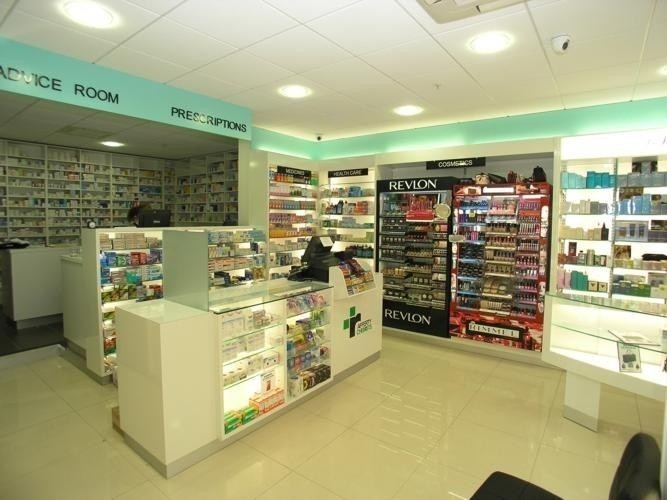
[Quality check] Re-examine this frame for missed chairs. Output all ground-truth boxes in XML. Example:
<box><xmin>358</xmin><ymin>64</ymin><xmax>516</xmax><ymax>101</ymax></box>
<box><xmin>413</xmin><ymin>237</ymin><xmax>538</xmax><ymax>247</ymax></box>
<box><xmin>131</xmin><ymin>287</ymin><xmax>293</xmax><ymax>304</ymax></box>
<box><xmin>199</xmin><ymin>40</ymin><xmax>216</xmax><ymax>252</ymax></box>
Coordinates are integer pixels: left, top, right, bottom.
<box><xmin>470</xmin><ymin>433</ymin><xmax>660</xmax><ymax>500</ymax></box>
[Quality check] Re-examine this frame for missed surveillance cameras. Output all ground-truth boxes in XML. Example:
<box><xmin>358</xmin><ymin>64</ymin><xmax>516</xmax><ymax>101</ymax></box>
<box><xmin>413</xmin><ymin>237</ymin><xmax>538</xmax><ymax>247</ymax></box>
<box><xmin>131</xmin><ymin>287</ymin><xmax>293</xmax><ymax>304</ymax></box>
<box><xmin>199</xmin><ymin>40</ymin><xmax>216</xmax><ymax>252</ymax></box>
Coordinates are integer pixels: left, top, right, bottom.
<box><xmin>551</xmin><ymin>35</ymin><xmax>571</xmax><ymax>54</ymax></box>
<box><xmin>316</xmin><ymin>134</ymin><xmax>325</xmax><ymax>142</ymax></box>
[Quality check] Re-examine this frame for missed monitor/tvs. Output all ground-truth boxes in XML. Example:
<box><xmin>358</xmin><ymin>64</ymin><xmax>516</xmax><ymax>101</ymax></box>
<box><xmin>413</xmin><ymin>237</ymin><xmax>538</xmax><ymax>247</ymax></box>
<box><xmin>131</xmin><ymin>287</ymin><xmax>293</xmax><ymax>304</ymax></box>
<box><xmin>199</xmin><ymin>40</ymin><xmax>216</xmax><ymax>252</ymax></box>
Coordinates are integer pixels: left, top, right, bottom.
<box><xmin>301</xmin><ymin>235</ymin><xmax>335</xmax><ymax>264</ymax></box>
<box><xmin>138</xmin><ymin>208</ymin><xmax>171</xmax><ymax>228</ymax></box>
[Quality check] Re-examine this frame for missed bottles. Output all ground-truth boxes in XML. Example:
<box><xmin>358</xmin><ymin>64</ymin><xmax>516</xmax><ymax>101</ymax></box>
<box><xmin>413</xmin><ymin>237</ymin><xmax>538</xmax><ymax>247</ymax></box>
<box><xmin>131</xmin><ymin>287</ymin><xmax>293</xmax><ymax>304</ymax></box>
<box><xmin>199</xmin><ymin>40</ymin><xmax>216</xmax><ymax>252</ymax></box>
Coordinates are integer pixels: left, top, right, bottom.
<box><xmin>457</xmin><ymin>200</ymin><xmax>541</xmax><ymax>317</ymax></box>
<box><xmin>321</xmin><ymin>186</ymin><xmax>350</xmax><ymax>214</ymax></box>
<box><xmin>345</xmin><ymin>243</ymin><xmax>374</xmax><ymax>260</ymax></box>
<box><xmin>268</xmin><ymin>170</ymin><xmax>318</xmax><ymax>238</ymax></box>
<box><xmin>381</xmin><ymin>195</ymin><xmax>434</xmax><ymax>301</ymax></box>
